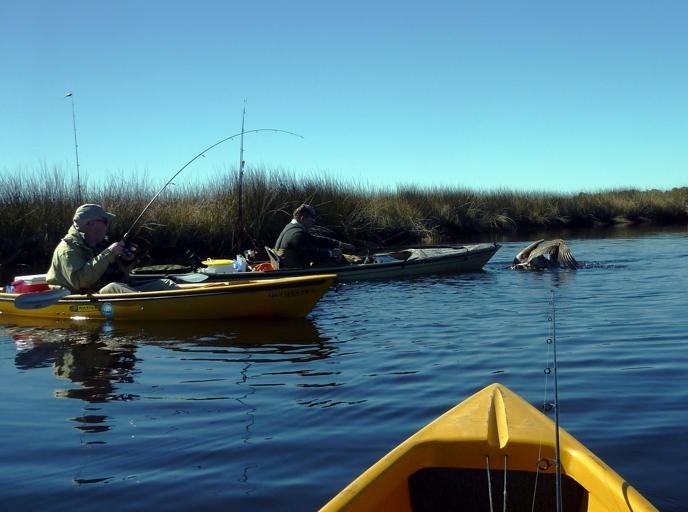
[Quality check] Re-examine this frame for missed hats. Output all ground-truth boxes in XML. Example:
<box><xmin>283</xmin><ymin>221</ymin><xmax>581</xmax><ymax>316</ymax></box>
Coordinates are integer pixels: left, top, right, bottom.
<box><xmin>72</xmin><ymin>204</ymin><xmax>116</xmax><ymax>232</ymax></box>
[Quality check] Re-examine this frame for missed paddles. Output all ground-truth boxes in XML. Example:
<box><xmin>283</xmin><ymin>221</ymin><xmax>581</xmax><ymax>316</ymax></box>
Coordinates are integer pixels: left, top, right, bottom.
<box><xmin>15</xmin><ymin>272</ymin><xmax>208</xmax><ymax>309</ymax></box>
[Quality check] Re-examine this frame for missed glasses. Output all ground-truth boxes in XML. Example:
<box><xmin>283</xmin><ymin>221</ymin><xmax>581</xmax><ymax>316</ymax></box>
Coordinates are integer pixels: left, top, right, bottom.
<box><xmin>90</xmin><ymin>218</ymin><xmax>108</xmax><ymax>227</ymax></box>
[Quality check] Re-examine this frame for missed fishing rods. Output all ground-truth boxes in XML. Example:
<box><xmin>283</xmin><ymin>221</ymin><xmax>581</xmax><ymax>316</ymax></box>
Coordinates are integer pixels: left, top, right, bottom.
<box><xmin>65</xmin><ymin>92</ymin><xmax>82</xmax><ymax>206</ymax></box>
<box><xmin>119</xmin><ymin>129</ymin><xmax>304</xmax><ymax>260</ymax></box>
<box><xmin>537</xmin><ymin>290</ymin><xmax>562</xmax><ymax>512</ymax></box>
<box><xmin>239</xmin><ymin>97</ymin><xmax>247</xmax><ymax>255</ymax></box>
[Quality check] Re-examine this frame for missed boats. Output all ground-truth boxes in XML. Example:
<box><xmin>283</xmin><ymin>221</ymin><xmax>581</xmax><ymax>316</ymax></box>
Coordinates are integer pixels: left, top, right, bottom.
<box><xmin>0</xmin><ymin>271</ymin><xmax>337</xmax><ymax>325</ymax></box>
<box><xmin>315</xmin><ymin>382</ymin><xmax>662</xmax><ymax>512</ymax></box>
<box><xmin>198</xmin><ymin>241</ymin><xmax>503</xmax><ymax>284</ymax></box>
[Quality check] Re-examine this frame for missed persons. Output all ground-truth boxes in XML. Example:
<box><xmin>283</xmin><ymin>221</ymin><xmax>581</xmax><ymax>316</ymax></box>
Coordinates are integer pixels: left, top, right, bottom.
<box><xmin>44</xmin><ymin>202</ymin><xmax>182</xmax><ymax>294</ymax></box>
<box><xmin>273</xmin><ymin>201</ymin><xmax>357</xmax><ymax>267</ymax></box>
